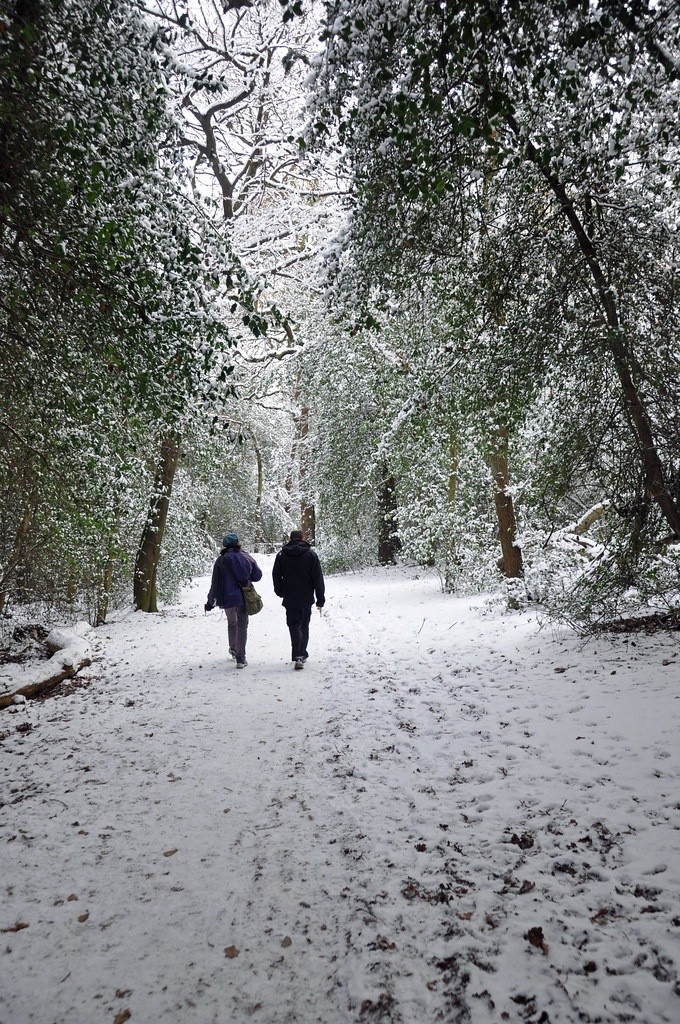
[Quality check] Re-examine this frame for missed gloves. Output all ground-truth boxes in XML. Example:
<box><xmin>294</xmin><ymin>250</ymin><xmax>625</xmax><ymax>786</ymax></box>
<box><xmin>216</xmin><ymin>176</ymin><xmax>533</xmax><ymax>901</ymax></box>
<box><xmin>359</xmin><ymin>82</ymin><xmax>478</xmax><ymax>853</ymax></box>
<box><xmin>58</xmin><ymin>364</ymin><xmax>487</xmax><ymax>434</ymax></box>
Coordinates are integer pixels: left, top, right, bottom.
<box><xmin>205</xmin><ymin>602</ymin><xmax>214</xmax><ymax>611</ymax></box>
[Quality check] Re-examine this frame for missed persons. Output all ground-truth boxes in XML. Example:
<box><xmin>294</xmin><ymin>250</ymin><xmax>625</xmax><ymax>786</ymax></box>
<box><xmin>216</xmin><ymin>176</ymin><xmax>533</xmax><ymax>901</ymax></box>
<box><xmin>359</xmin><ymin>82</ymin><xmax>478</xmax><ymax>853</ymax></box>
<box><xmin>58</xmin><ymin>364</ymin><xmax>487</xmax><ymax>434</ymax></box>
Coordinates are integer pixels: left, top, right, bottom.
<box><xmin>272</xmin><ymin>531</ymin><xmax>325</xmax><ymax>669</ymax></box>
<box><xmin>205</xmin><ymin>534</ymin><xmax>263</xmax><ymax>669</ymax></box>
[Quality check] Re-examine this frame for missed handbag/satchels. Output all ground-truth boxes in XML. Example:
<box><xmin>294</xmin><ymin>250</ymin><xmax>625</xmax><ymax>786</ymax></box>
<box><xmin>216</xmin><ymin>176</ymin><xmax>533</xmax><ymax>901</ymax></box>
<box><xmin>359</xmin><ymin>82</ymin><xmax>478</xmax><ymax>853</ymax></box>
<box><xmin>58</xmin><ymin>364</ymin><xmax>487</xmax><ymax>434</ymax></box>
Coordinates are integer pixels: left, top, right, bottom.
<box><xmin>241</xmin><ymin>586</ymin><xmax>263</xmax><ymax>615</ymax></box>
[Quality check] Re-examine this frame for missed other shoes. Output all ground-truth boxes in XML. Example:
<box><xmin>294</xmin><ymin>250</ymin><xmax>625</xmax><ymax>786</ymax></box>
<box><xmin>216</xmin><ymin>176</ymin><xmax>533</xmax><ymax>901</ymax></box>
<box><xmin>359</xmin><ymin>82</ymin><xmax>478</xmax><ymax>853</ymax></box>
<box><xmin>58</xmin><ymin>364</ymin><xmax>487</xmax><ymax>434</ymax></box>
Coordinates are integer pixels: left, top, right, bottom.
<box><xmin>295</xmin><ymin>656</ymin><xmax>306</xmax><ymax>669</ymax></box>
<box><xmin>229</xmin><ymin>647</ymin><xmax>236</xmax><ymax>659</ymax></box>
<box><xmin>237</xmin><ymin>660</ymin><xmax>248</xmax><ymax>668</ymax></box>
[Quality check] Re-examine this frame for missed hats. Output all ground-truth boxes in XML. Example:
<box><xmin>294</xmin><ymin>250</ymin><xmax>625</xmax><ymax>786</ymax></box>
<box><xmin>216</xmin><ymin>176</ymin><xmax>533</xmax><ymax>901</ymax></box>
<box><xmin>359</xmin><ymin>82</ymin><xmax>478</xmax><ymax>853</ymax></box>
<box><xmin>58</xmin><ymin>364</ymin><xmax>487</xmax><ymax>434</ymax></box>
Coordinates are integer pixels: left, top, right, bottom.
<box><xmin>223</xmin><ymin>534</ymin><xmax>238</xmax><ymax>546</ymax></box>
